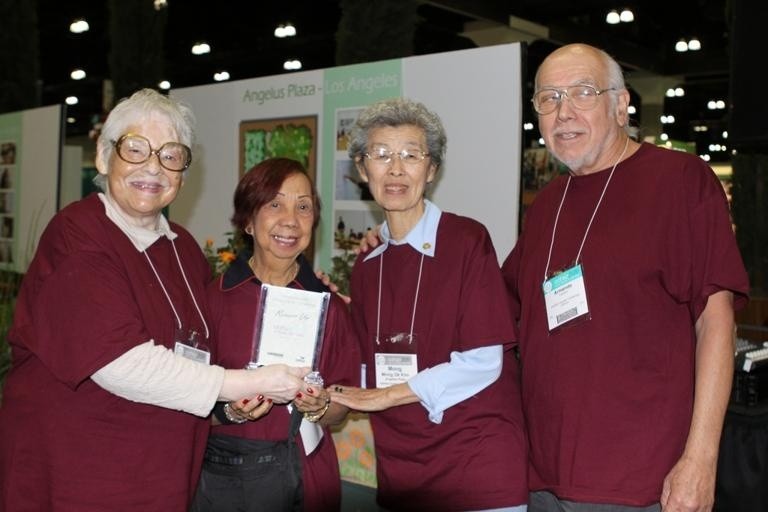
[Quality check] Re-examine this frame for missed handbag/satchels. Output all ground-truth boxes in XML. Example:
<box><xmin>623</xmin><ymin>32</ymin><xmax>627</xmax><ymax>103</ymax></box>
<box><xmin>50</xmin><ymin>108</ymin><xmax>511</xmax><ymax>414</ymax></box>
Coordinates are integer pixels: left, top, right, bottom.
<box><xmin>189</xmin><ymin>435</ymin><xmax>303</xmax><ymax>512</ymax></box>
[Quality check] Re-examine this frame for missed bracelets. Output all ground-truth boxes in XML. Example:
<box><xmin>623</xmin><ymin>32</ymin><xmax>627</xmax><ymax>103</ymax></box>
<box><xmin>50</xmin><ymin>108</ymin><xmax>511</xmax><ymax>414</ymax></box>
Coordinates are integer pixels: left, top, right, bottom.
<box><xmin>301</xmin><ymin>400</ymin><xmax>331</xmax><ymax>423</ymax></box>
<box><xmin>223</xmin><ymin>400</ymin><xmax>248</xmax><ymax>428</ymax></box>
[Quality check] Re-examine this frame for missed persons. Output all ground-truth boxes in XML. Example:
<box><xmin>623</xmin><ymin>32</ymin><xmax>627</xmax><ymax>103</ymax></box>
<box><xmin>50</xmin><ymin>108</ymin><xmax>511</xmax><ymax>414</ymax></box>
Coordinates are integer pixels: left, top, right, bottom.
<box><xmin>1</xmin><ymin>87</ymin><xmax>321</xmax><ymax>511</ymax></box>
<box><xmin>199</xmin><ymin>156</ymin><xmax>362</xmax><ymax>509</ymax></box>
<box><xmin>325</xmin><ymin>95</ymin><xmax>533</xmax><ymax>511</ymax></box>
<box><xmin>357</xmin><ymin>42</ymin><xmax>754</xmax><ymax>511</ymax></box>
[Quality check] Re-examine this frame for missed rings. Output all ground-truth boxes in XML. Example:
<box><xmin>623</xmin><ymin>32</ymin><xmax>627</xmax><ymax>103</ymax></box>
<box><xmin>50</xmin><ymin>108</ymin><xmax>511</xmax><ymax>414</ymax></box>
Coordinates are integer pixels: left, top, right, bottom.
<box><xmin>334</xmin><ymin>386</ymin><xmax>344</xmax><ymax>393</ymax></box>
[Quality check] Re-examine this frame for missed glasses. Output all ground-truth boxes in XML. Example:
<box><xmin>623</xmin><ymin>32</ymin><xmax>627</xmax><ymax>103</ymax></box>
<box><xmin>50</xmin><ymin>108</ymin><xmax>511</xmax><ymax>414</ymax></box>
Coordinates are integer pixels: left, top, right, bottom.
<box><xmin>361</xmin><ymin>147</ymin><xmax>430</xmax><ymax>164</ymax></box>
<box><xmin>110</xmin><ymin>133</ymin><xmax>191</xmax><ymax>171</ymax></box>
<box><xmin>531</xmin><ymin>84</ymin><xmax>616</xmax><ymax>115</ymax></box>
